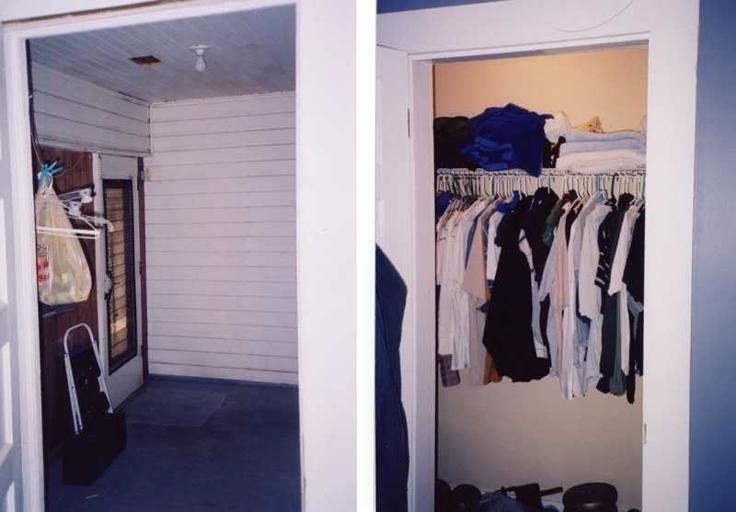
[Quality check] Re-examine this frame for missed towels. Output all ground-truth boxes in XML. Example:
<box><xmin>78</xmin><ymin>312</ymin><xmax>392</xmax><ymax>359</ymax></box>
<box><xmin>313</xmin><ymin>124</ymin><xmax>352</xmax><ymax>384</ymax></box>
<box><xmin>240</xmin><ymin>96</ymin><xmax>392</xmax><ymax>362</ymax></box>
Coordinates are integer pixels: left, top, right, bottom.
<box><xmin>556</xmin><ymin>127</ymin><xmax>645</xmax><ymax>171</ymax></box>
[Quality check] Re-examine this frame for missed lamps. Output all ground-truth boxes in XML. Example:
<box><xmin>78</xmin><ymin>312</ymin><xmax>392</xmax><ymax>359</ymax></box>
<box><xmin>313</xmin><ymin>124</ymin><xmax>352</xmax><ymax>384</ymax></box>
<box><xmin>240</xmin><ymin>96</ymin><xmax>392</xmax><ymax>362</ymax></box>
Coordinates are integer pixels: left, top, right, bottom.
<box><xmin>191</xmin><ymin>44</ymin><xmax>211</xmax><ymax>73</ymax></box>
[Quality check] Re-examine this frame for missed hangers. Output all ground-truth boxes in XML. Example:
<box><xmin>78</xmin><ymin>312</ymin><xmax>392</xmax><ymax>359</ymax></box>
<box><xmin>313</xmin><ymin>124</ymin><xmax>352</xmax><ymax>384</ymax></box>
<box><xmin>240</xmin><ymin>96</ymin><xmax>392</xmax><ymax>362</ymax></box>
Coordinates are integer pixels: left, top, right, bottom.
<box><xmin>34</xmin><ymin>171</ymin><xmax>115</xmax><ymax>240</ymax></box>
<box><xmin>436</xmin><ymin>168</ymin><xmax>645</xmax><ymax>214</ymax></box>
